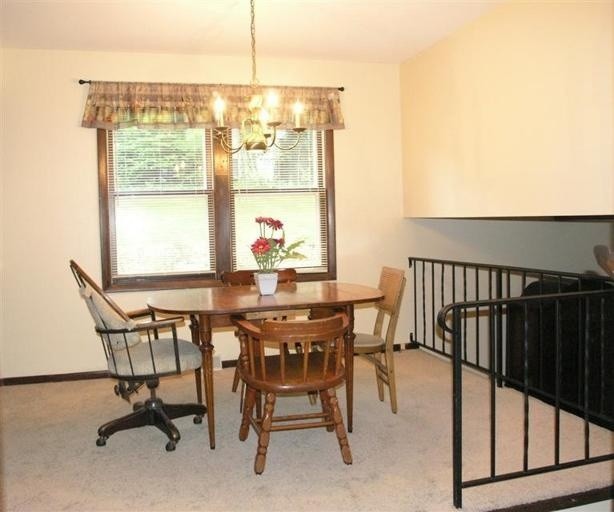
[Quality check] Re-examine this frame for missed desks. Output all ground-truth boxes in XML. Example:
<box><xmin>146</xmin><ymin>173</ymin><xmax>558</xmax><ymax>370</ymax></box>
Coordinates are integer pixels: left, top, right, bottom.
<box><xmin>146</xmin><ymin>280</ymin><xmax>386</xmax><ymax>449</ymax></box>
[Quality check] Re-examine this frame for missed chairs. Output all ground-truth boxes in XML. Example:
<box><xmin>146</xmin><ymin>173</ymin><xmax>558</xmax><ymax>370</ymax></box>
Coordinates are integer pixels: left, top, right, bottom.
<box><xmin>329</xmin><ymin>265</ymin><xmax>407</xmax><ymax>413</ymax></box>
<box><xmin>230</xmin><ymin>307</ymin><xmax>354</xmax><ymax>474</ymax></box>
<box><xmin>68</xmin><ymin>260</ymin><xmax>208</xmax><ymax>452</ymax></box>
<box><xmin>220</xmin><ymin>268</ymin><xmax>317</xmax><ymax>405</ymax></box>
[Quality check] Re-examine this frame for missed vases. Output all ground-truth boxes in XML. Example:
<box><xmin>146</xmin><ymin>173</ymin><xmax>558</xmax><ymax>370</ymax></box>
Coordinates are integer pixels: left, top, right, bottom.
<box><xmin>254</xmin><ymin>272</ymin><xmax>279</xmax><ymax>296</ymax></box>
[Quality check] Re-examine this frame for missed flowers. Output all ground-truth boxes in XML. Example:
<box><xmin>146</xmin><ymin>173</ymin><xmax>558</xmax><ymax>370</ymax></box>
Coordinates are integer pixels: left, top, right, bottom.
<box><xmin>251</xmin><ymin>218</ymin><xmax>306</xmax><ymax>274</ymax></box>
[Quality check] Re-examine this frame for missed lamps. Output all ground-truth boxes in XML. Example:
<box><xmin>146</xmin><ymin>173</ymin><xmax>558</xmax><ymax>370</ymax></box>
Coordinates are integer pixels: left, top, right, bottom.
<box><xmin>217</xmin><ymin>0</ymin><xmax>306</xmax><ymax>153</ymax></box>
